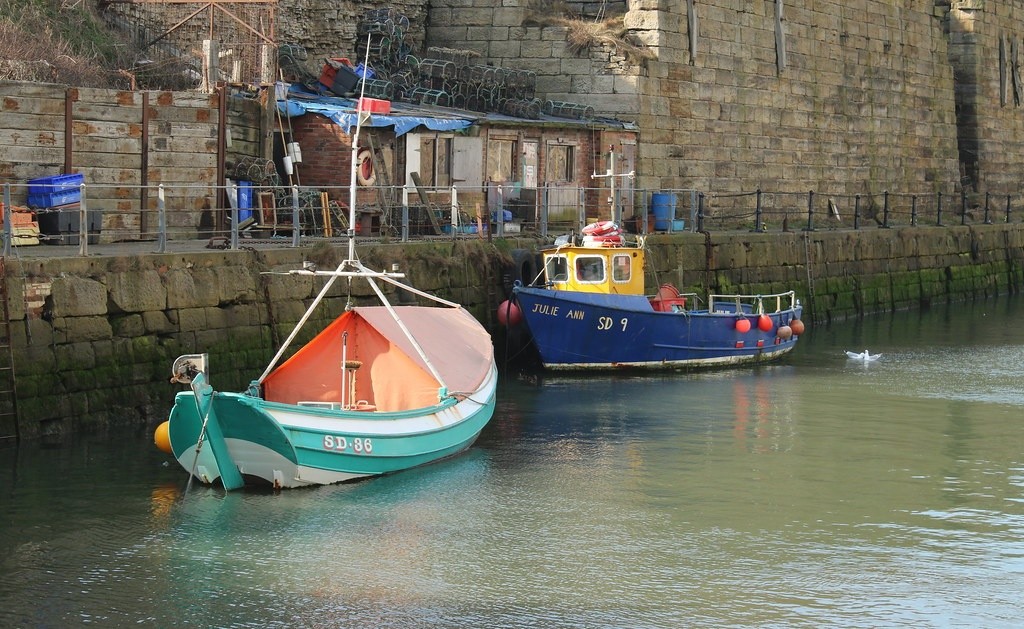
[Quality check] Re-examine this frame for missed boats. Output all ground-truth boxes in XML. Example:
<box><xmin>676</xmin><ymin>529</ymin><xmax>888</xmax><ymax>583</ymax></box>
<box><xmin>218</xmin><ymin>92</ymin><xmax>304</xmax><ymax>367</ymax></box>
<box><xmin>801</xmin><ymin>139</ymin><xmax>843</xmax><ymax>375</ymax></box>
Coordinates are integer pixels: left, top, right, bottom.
<box><xmin>155</xmin><ymin>33</ymin><xmax>498</xmax><ymax>488</ymax></box>
<box><xmin>498</xmin><ymin>144</ymin><xmax>804</xmax><ymax>369</ymax></box>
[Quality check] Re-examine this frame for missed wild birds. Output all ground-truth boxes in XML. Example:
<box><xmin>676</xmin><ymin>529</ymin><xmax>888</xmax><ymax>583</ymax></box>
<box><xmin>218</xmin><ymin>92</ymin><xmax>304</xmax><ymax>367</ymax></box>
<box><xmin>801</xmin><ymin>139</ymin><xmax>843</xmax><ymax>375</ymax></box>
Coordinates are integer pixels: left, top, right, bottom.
<box><xmin>864</xmin><ymin>349</ymin><xmax>883</xmax><ymax>360</ymax></box>
<box><xmin>842</xmin><ymin>349</ymin><xmax>864</xmax><ymax>360</ymax></box>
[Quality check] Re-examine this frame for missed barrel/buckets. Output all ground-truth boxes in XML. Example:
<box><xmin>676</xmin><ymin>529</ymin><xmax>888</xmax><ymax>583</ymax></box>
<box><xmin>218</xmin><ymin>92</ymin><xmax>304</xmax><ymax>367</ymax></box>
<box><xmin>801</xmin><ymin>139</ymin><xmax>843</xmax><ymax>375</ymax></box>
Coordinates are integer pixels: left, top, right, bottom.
<box><xmin>494</xmin><ymin>210</ymin><xmax>512</xmax><ymax>222</ymax></box>
<box><xmin>342</xmin><ymin>400</ymin><xmax>376</xmax><ymax>412</ymax></box>
<box><xmin>655</xmin><ymin>284</ymin><xmax>679</xmax><ymax>298</ymax></box>
<box><xmin>652</xmin><ymin>193</ymin><xmax>676</xmax><ymax>230</ymax></box>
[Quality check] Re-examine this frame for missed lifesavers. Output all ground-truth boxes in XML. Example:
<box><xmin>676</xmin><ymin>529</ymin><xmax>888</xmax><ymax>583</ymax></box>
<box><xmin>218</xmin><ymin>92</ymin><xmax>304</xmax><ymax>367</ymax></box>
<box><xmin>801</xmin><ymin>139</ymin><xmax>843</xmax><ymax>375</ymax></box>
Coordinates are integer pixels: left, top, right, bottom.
<box><xmin>581</xmin><ymin>221</ymin><xmax>625</xmax><ymax>246</ymax></box>
<box><xmin>357</xmin><ymin>150</ymin><xmax>377</xmax><ymax>186</ymax></box>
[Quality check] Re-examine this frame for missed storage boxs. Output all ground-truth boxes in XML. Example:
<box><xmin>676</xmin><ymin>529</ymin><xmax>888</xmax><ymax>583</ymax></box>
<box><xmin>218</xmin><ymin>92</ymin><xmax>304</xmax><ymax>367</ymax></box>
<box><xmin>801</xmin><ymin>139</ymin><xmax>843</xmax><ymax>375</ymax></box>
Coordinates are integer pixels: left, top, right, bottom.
<box><xmin>28</xmin><ymin>187</ymin><xmax>80</xmax><ymax>208</ymax></box>
<box><xmin>238</xmin><ymin>180</ymin><xmax>253</xmax><ymax>223</ymax></box>
<box><xmin>332</xmin><ymin>64</ymin><xmax>361</xmax><ymax>97</ymax></box>
<box><xmin>355</xmin><ymin>62</ymin><xmax>376</xmax><ymax>80</ymax></box>
<box><xmin>26</xmin><ymin>172</ymin><xmax>84</xmax><ymax>194</ymax></box>
<box><xmin>648</xmin><ymin>296</ymin><xmax>687</xmax><ymax>312</ymax></box>
<box><xmin>357</xmin><ymin>96</ymin><xmax>390</xmax><ymax>116</ymax></box>
<box><xmin>275</xmin><ymin>81</ymin><xmax>292</xmax><ymax>102</ymax></box>
<box><xmin>37</xmin><ymin>208</ymin><xmax>102</xmax><ymax>244</ymax></box>
<box><xmin>712</xmin><ymin>301</ymin><xmax>753</xmax><ymax>314</ymax></box>
<box><xmin>318</xmin><ymin>56</ymin><xmax>354</xmax><ymax>88</ymax></box>
<box><xmin>667</xmin><ymin>218</ymin><xmax>686</xmax><ymax>231</ymax></box>
